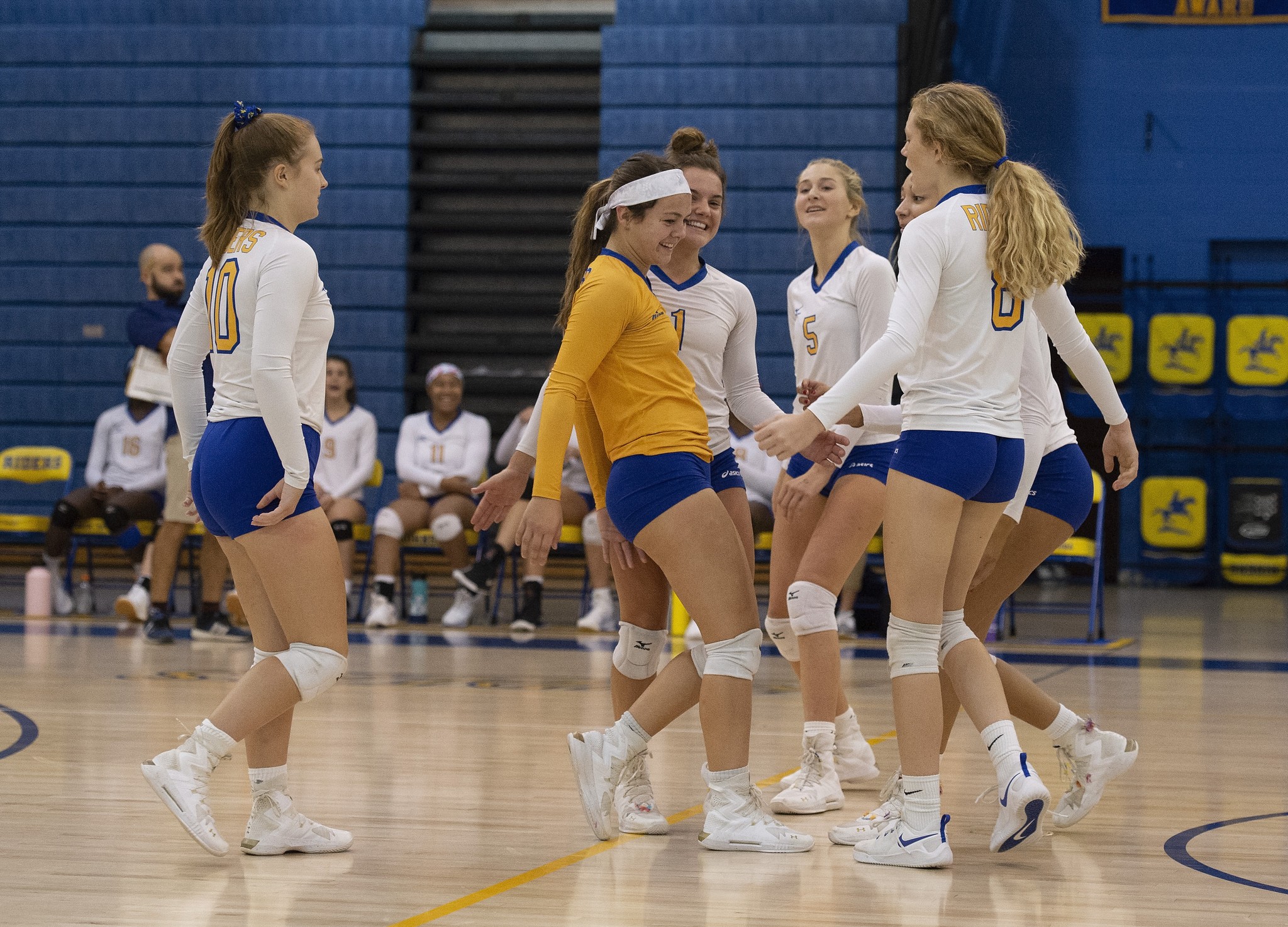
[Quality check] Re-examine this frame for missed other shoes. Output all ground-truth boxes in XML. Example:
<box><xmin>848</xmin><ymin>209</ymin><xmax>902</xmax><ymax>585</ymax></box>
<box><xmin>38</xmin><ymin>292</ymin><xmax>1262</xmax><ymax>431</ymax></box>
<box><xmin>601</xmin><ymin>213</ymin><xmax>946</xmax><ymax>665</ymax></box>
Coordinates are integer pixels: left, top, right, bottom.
<box><xmin>42</xmin><ymin>570</ymin><xmax>73</xmax><ymax>616</ymax></box>
<box><xmin>852</xmin><ymin>863</ymin><xmax>953</xmax><ymax>926</ymax></box>
<box><xmin>115</xmin><ymin>583</ymin><xmax>148</xmax><ymax>622</ymax></box>
<box><xmin>702</xmin><ymin>854</ymin><xmax>800</xmax><ymax>925</ymax></box>
<box><xmin>241</xmin><ymin>857</ymin><xmax>354</xmax><ymax>914</ymax></box>
<box><xmin>578</xmin><ymin>605</ymin><xmax>616</xmax><ymax>633</ymax></box>
<box><xmin>566</xmin><ymin>837</ymin><xmax>669</xmax><ymax>922</ymax></box>
<box><xmin>121</xmin><ymin>866</ymin><xmax>226</xmax><ymax>920</ymax></box>
<box><xmin>835</xmin><ymin>610</ymin><xmax>857</xmax><ymax>639</ymax></box>
<box><xmin>441</xmin><ymin>588</ymin><xmax>480</xmax><ymax>628</ymax></box>
<box><xmin>510</xmin><ymin>599</ymin><xmax>541</xmax><ymax>631</ymax></box>
<box><xmin>684</xmin><ymin>620</ymin><xmax>704</xmax><ymax>641</ymax></box>
<box><xmin>452</xmin><ymin>561</ymin><xmax>497</xmax><ymax>597</ymax></box>
<box><xmin>190</xmin><ymin>611</ymin><xmax>251</xmax><ymax>643</ymax></box>
<box><xmin>365</xmin><ymin>594</ymin><xmax>398</xmax><ymax>628</ymax></box>
<box><xmin>1050</xmin><ymin>835</ymin><xmax>1136</xmax><ymax>925</ymax></box>
<box><xmin>141</xmin><ymin>607</ymin><xmax>174</xmax><ymax>645</ymax></box>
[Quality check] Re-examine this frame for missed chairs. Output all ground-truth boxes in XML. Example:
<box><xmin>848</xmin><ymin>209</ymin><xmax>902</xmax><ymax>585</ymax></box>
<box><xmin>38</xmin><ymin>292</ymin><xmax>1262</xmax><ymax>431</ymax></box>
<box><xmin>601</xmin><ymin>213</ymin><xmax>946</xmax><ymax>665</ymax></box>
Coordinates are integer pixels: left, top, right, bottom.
<box><xmin>0</xmin><ymin>443</ymin><xmax>1104</xmax><ymax>640</ymax></box>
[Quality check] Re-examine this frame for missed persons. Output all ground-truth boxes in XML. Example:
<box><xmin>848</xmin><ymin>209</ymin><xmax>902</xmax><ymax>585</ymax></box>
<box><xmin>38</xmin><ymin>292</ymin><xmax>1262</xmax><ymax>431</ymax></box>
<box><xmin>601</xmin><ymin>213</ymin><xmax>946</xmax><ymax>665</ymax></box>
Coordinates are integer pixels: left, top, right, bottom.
<box><xmin>140</xmin><ymin>100</ymin><xmax>354</xmax><ymax>857</ymax></box>
<box><xmin>123</xmin><ymin>242</ymin><xmax>253</xmax><ymax>647</ymax></box>
<box><xmin>473</xmin><ymin>127</ymin><xmax>851</xmax><ymax>836</ymax></box>
<box><xmin>307</xmin><ymin>163</ymin><xmax>1138</xmax><ymax>827</ymax></box>
<box><xmin>751</xmin><ymin>81</ymin><xmax>1139</xmax><ymax>868</ymax></box>
<box><xmin>513</xmin><ymin>151</ymin><xmax>816</xmax><ymax>853</ymax></box>
<box><xmin>44</xmin><ymin>354</ymin><xmax>254</xmax><ymax>630</ymax></box>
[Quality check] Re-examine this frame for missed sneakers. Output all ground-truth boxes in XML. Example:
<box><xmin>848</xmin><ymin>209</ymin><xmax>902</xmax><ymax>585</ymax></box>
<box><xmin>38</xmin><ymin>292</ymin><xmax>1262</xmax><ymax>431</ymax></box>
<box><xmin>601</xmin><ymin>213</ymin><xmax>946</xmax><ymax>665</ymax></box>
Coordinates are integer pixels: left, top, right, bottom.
<box><xmin>614</xmin><ymin>749</ymin><xmax>670</xmax><ymax>835</ymax></box>
<box><xmin>828</xmin><ymin>770</ymin><xmax>905</xmax><ymax>845</ymax></box>
<box><xmin>1052</xmin><ymin>713</ymin><xmax>1139</xmax><ymax>828</ymax></box>
<box><xmin>698</xmin><ymin>771</ymin><xmax>815</xmax><ymax>853</ymax></box>
<box><xmin>780</xmin><ymin>716</ymin><xmax>881</xmax><ymax>791</ymax></box>
<box><xmin>567</xmin><ymin>720</ymin><xmax>649</xmax><ymax>840</ymax></box>
<box><xmin>852</xmin><ymin>807</ymin><xmax>953</xmax><ymax>868</ymax></box>
<box><xmin>240</xmin><ymin>771</ymin><xmax>353</xmax><ymax>856</ymax></box>
<box><xmin>975</xmin><ymin>752</ymin><xmax>1051</xmax><ymax>853</ymax></box>
<box><xmin>770</xmin><ymin>732</ymin><xmax>846</xmax><ymax>815</ymax></box>
<box><xmin>140</xmin><ymin>718</ymin><xmax>233</xmax><ymax>857</ymax></box>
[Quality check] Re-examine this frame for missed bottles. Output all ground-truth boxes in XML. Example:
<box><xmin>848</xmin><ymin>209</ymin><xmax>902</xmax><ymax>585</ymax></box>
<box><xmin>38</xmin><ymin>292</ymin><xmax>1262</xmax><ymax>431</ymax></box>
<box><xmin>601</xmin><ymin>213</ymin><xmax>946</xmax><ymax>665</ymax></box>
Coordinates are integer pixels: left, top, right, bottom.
<box><xmin>24</xmin><ymin>561</ymin><xmax>51</xmax><ymax>617</ymax></box>
<box><xmin>409</xmin><ymin>573</ymin><xmax>429</xmax><ymax>624</ymax></box>
<box><xmin>76</xmin><ymin>574</ymin><xmax>91</xmax><ymax>615</ymax></box>
